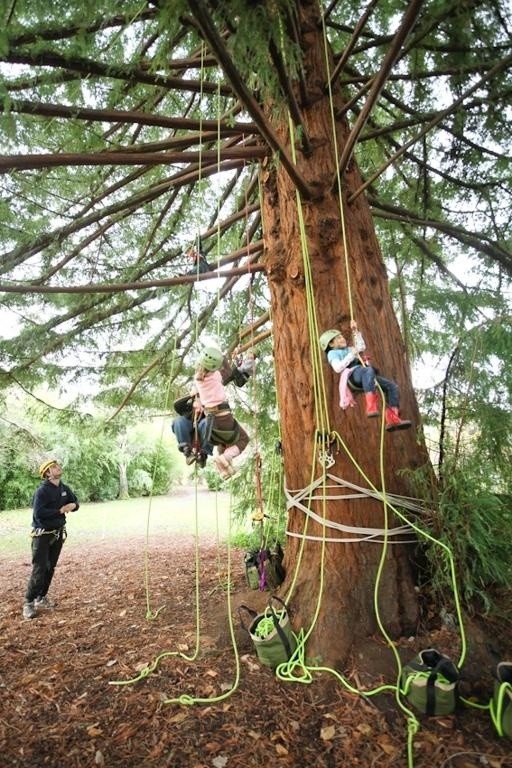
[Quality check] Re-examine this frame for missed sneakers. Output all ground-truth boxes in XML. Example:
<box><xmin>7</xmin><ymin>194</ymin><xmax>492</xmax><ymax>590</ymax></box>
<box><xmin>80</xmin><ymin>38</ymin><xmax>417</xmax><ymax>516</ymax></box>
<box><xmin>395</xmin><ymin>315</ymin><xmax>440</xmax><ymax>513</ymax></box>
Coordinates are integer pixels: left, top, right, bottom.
<box><xmin>186</xmin><ymin>453</ymin><xmax>195</xmax><ymax>466</ymax></box>
<box><xmin>23</xmin><ymin>602</ymin><xmax>36</xmax><ymax>617</ymax></box>
<box><xmin>197</xmin><ymin>451</ymin><xmax>207</xmax><ymax>468</ymax></box>
<box><xmin>34</xmin><ymin>596</ymin><xmax>55</xmax><ymax>608</ymax></box>
<box><xmin>215</xmin><ymin>458</ymin><xmax>231</xmax><ymax>481</ymax></box>
<box><xmin>219</xmin><ymin>455</ymin><xmax>236</xmax><ymax>476</ymax></box>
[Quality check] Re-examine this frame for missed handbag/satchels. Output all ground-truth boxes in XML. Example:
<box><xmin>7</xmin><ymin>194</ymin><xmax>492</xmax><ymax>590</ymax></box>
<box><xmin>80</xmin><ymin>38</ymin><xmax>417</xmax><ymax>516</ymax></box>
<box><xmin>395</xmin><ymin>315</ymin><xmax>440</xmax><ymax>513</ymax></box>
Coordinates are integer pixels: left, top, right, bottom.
<box><xmin>402</xmin><ymin>650</ymin><xmax>460</xmax><ymax>715</ymax></box>
<box><xmin>266</xmin><ymin>559</ymin><xmax>285</xmax><ymax>588</ymax></box>
<box><xmin>492</xmin><ymin>662</ymin><xmax>512</xmax><ymax>738</ymax></box>
<box><xmin>238</xmin><ymin>596</ymin><xmax>297</xmax><ymax>668</ymax></box>
<box><xmin>244</xmin><ymin>553</ymin><xmax>260</xmax><ymax>589</ymax></box>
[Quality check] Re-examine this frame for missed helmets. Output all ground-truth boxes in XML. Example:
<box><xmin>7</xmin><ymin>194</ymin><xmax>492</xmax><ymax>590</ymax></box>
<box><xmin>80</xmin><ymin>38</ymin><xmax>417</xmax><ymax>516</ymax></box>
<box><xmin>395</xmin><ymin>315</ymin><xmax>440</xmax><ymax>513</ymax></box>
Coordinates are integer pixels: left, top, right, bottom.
<box><xmin>247</xmin><ymin>348</ymin><xmax>260</xmax><ymax>358</ymax></box>
<box><xmin>181</xmin><ymin>243</ymin><xmax>192</xmax><ymax>253</ymax></box>
<box><xmin>40</xmin><ymin>460</ymin><xmax>56</xmax><ymax>480</ymax></box>
<box><xmin>198</xmin><ymin>347</ymin><xmax>224</xmax><ymax>372</ymax></box>
<box><xmin>320</xmin><ymin>330</ymin><xmax>342</xmax><ymax>352</ymax></box>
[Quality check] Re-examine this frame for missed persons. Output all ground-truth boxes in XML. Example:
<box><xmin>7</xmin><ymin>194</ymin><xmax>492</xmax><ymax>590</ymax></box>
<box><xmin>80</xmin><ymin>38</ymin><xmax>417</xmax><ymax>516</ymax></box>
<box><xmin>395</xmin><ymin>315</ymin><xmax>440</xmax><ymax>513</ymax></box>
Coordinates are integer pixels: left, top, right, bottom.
<box><xmin>194</xmin><ymin>347</ymin><xmax>251</xmax><ymax>482</ymax></box>
<box><xmin>171</xmin><ymin>393</ymin><xmax>216</xmax><ymax>470</ymax></box>
<box><xmin>222</xmin><ymin>348</ymin><xmax>260</xmax><ymax>387</ymax></box>
<box><xmin>319</xmin><ymin>329</ymin><xmax>412</xmax><ymax>433</ymax></box>
<box><xmin>23</xmin><ymin>460</ymin><xmax>79</xmax><ymax>619</ymax></box>
<box><xmin>174</xmin><ymin>243</ymin><xmax>208</xmax><ymax>278</ymax></box>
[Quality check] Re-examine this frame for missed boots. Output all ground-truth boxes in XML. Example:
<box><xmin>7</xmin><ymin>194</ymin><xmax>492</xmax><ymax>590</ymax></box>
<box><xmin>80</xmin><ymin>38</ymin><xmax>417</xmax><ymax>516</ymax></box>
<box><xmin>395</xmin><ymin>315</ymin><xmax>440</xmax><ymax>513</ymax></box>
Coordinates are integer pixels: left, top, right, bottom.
<box><xmin>366</xmin><ymin>392</ymin><xmax>380</xmax><ymax>417</ymax></box>
<box><xmin>385</xmin><ymin>406</ymin><xmax>411</xmax><ymax>432</ymax></box>
